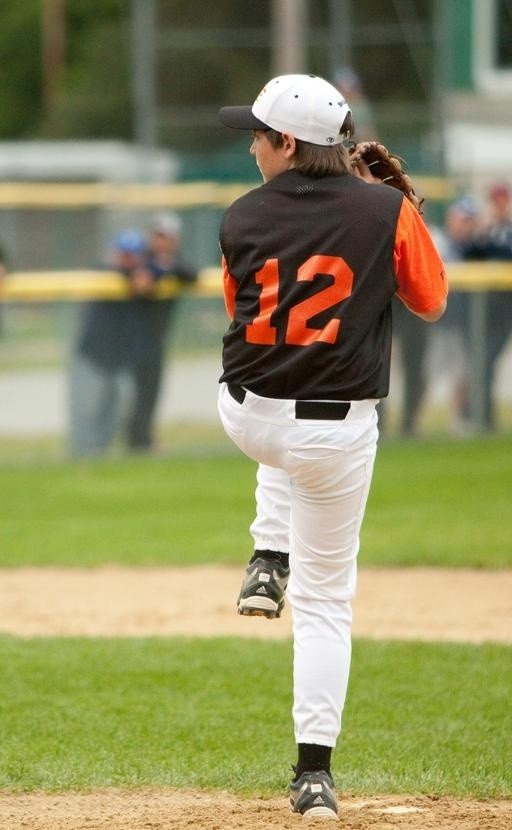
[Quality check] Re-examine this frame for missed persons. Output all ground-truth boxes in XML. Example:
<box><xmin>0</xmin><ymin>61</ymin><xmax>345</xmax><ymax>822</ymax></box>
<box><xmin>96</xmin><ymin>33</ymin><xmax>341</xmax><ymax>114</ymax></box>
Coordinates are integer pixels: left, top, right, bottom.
<box><xmin>67</xmin><ymin>227</ymin><xmax>159</xmax><ymax>469</ymax></box>
<box><xmin>120</xmin><ymin>208</ymin><xmax>201</xmax><ymax>457</ymax></box>
<box><xmin>215</xmin><ymin>72</ymin><xmax>450</xmax><ymax>822</ymax></box>
<box><xmin>453</xmin><ymin>179</ymin><xmax>511</xmax><ymax>438</ymax></box>
<box><xmin>376</xmin><ymin>194</ymin><xmax>485</xmax><ymax>437</ymax></box>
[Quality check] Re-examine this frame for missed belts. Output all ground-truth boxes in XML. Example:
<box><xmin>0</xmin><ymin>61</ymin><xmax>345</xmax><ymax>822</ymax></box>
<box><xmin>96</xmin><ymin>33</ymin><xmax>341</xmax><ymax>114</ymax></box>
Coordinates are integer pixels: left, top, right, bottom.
<box><xmin>227</xmin><ymin>384</ymin><xmax>351</xmax><ymax>420</ymax></box>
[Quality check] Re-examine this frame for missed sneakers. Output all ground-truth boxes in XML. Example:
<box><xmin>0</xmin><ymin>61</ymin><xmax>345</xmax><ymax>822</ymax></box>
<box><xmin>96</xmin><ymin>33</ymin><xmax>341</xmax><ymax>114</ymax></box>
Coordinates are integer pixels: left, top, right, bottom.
<box><xmin>289</xmin><ymin>770</ymin><xmax>341</xmax><ymax>824</ymax></box>
<box><xmin>237</xmin><ymin>559</ymin><xmax>290</xmax><ymax>619</ymax></box>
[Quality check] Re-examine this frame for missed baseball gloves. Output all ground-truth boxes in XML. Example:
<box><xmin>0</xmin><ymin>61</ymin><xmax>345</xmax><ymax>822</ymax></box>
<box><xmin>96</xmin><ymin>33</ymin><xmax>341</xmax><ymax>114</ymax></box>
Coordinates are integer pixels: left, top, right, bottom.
<box><xmin>348</xmin><ymin>142</ymin><xmax>426</xmax><ymax>216</ymax></box>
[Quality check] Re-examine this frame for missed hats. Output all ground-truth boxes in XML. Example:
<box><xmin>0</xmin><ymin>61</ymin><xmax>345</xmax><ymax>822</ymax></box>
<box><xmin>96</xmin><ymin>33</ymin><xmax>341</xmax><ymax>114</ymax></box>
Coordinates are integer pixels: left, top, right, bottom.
<box><xmin>150</xmin><ymin>213</ymin><xmax>182</xmax><ymax>239</ymax></box>
<box><xmin>117</xmin><ymin>230</ymin><xmax>145</xmax><ymax>254</ymax></box>
<box><xmin>217</xmin><ymin>74</ymin><xmax>353</xmax><ymax>146</ymax></box>
